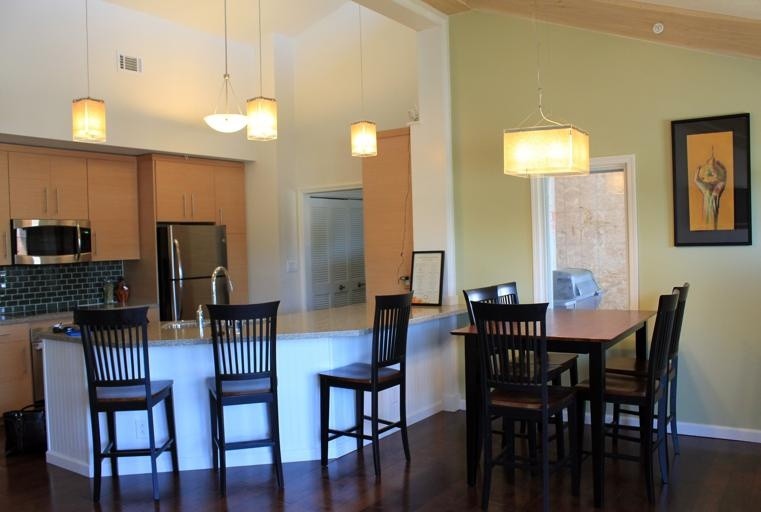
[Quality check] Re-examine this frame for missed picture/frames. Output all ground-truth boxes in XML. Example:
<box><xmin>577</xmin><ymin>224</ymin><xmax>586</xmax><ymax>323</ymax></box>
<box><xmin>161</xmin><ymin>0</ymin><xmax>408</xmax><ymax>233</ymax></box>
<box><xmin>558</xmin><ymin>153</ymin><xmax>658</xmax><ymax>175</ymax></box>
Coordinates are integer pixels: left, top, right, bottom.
<box><xmin>409</xmin><ymin>251</ymin><xmax>445</xmax><ymax>308</ymax></box>
<box><xmin>672</xmin><ymin>112</ymin><xmax>754</xmax><ymax>248</ymax></box>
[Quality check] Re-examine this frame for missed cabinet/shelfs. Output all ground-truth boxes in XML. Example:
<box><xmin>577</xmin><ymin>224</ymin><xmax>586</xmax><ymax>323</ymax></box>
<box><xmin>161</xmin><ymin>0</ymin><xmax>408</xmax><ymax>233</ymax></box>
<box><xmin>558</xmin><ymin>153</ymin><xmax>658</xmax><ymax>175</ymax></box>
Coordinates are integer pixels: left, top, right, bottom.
<box><xmin>0</xmin><ymin>323</ymin><xmax>36</xmax><ymax>421</ymax></box>
<box><xmin>154</xmin><ymin>156</ymin><xmax>215</xmax><ymax>224</ymax></box>
<box><xmin>8</xmin><ymin>142</ymin><xmax>89</xmax><ymax>220</ymax></box>
<box><xmin>216</xmin><ymin>155</ymin><xmax>252</xmax><ymax>322</ymax></box>
<box><xmin>87</xmin><ymin>152</ymin><xmax>143</xmax><ymax>261</ymax></box>
<box><xmin>300</xmin><ymin>189</ymin><xmax>365</xmax><ymax>310</ymax></box>
<box><xmin>30</xmin><ymin>319</ymin><xmax>77</xmax><ymax>403</ymax></box>
<box><xmin>0</xmin><ymin>143</ymin><xmax>9</xmax><ymax>268</ymax></box>
<box><xmin>362</xmin><ymin>127</ymin><xmax>413</xmax><ymax>313</ymax></box>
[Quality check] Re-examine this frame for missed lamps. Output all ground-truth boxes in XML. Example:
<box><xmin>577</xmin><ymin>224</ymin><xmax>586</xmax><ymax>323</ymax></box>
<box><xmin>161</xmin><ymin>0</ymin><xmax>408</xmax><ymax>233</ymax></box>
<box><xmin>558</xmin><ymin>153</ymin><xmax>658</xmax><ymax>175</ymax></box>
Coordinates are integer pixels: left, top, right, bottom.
<box><xmin>205</xmin><ymin>2</ymin><xmax>248</xmax><ymax>134</ymax></box>
<box><xmin>350</xmin><ymin>6</ymin><xmax>379</xmax><ymax>158</ymax></box>
<box><xmin>73</xmin><ymin>1</ymin><xmax>108</xmax><ymax>143</ymax></box>
<box><xmin>504</xmin><ymin>12</ymin><xmax>590</xmax><ymax>179</ymax></box>
<box><xmin>248</xmin><ymin>1</ymin><xmax>278</xmax><ymax>142</ymax></box>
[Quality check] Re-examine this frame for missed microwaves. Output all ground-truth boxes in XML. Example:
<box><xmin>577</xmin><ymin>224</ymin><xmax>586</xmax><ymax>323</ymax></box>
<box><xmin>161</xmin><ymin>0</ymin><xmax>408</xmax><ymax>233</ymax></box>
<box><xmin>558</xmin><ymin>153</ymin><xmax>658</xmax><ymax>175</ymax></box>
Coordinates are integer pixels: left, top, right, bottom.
<box><xmin>11</xmin><ymin>219</ymin><xmax>97</xmax><ymax>264</ymax></box>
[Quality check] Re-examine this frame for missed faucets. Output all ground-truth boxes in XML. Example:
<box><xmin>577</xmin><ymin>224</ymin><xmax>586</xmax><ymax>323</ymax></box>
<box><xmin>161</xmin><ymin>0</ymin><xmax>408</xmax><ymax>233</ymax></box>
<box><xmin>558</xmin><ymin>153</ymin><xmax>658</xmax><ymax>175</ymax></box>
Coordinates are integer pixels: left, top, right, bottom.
<box><xmin>210</xmin><ymin>266</ymin><xmax>233</xmax><ymax>336</ymax></box>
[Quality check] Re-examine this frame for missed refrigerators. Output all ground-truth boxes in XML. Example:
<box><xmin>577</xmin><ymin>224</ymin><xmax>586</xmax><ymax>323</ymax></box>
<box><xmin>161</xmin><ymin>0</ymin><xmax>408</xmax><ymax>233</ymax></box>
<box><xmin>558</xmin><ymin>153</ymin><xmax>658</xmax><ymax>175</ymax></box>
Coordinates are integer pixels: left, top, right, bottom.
<box><xmin>158</xmin><ymin>223</ymin><xmax>232</xmax><ymax>322</ymax></box>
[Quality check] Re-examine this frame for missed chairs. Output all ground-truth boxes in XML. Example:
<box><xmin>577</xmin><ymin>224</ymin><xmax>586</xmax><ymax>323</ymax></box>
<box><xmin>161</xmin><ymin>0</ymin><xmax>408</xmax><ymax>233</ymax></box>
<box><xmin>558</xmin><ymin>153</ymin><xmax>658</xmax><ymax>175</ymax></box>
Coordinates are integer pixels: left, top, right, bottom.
<box><xmin>499</xmin><ymin>282</ymin><xmax>583</xmax><ymax>441</ymax></box>
<box><xmin>206</xmin><ymin>301</ymin><xmax>283</xmax><ymax>481</ymax></box>
<box><xmin>464</xmin><ymin>286</ymin><xmax>565</xmax><ymax>457</ymax></box>
<box><xmin>577</xmin><ymin>290</ymin><xmax>669</xmax><ymax>506</ymax></box>
<box><xmin>320</xmin><ymin>291</ymin><xmax>415</xmax><ymax>476</ymax></box>
<box><xmin>469</xmin><ymin>302</ymin><xmax>580</xmax><ymax>512</ymax></box>
<box><xmin>608</xmin><ymin>281</ymin><xmax>690</xmax><ymax>459</ymax></box>
<box><xmin>74</xmin><ymin>304</ymin><xmax>181</xmax><ymax>502</ymax></box>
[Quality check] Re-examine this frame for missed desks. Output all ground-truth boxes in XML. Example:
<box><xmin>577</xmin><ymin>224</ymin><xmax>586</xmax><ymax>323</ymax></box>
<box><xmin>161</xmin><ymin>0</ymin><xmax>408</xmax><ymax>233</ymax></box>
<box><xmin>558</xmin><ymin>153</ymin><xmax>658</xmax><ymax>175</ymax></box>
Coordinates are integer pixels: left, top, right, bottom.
<box><xmin>450</xmin><ymin>309</ymin><xmax>658</xmax><ymax>507</ymax></box>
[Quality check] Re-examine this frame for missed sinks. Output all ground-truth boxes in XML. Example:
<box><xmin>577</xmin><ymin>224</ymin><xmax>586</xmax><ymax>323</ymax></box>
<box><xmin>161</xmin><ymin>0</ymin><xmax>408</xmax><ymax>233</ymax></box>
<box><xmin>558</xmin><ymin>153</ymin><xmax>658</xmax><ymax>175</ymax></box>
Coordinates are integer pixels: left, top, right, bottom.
<box><xmin>161</xmin><ymin>319</ymin><xmax>271</xmax><ymax>329</ymax></box>
